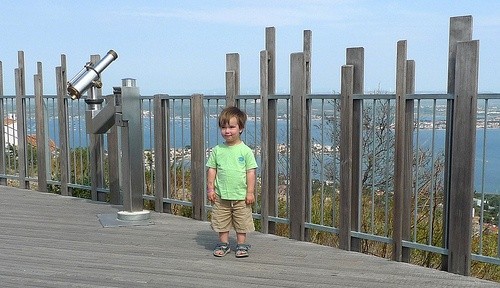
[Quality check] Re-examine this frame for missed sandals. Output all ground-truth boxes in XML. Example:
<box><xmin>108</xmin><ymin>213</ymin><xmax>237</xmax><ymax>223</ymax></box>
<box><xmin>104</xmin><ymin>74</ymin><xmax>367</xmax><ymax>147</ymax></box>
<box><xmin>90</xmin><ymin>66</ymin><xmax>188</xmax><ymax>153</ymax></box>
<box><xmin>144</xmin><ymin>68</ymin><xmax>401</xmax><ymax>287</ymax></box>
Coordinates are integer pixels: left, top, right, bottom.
<box><xmin>213</xmin><ymin>242</ymin><xmax>230</xmax><ymax>257</ymax></box>
<box><xmin>235</xmin><ymin>243</ymin><xmax>251</xmax><ymax>257</ymax></box>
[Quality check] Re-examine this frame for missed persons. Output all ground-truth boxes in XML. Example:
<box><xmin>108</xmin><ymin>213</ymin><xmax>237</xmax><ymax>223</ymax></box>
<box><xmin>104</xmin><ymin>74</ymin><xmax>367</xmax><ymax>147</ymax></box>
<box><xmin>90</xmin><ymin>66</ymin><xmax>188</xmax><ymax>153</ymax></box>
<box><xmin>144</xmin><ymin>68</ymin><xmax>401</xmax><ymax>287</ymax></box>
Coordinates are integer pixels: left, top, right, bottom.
<box><xmin>206</xmin><ymin>107</ymin><xmax>259</xmax><ymax>258</ymax></box>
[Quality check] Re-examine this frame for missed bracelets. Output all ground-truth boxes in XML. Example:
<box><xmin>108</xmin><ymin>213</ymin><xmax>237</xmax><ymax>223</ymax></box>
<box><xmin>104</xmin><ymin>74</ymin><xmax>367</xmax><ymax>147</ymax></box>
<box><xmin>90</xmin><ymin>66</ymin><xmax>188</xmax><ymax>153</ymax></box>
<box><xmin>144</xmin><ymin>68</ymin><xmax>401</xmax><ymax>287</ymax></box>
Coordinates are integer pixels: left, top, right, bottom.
<box><xmin>207</xmin><ymin>188</ymin><xmax>215</xmax><ymax>193</ymax></box>
